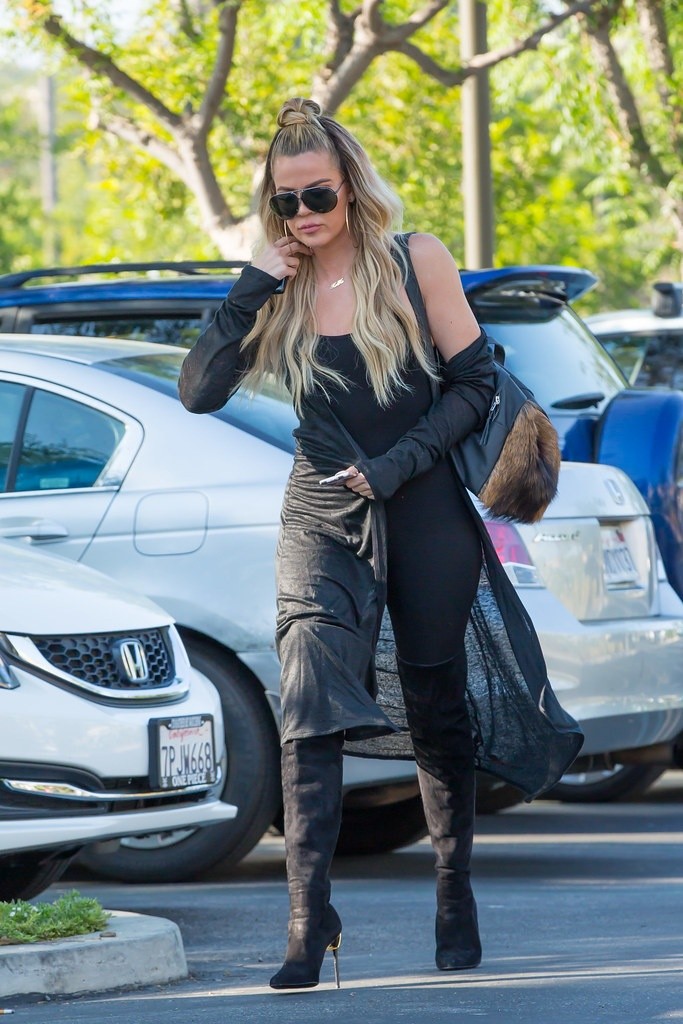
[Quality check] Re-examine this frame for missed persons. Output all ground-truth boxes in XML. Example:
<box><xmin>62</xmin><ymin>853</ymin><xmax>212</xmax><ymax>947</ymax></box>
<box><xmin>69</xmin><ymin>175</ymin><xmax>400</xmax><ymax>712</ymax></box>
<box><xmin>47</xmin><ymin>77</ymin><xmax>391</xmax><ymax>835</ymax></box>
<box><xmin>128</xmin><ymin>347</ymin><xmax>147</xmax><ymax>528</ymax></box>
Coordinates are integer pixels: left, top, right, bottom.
<box><xmin>177</xmin><ymin>95</ymin><xmax>502</xmax><ymax>992</ymax></box>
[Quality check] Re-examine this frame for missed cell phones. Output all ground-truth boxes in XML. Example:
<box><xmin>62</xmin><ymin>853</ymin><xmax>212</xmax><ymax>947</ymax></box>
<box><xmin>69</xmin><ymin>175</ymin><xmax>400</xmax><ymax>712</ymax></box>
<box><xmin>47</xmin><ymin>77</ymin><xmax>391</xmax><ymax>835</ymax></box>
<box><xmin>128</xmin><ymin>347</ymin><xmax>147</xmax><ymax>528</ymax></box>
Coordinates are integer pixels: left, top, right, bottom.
<box><xmin>320</xmin><ymin>471</ymin><xmax>359</xmax><ymax>487</ymax></box>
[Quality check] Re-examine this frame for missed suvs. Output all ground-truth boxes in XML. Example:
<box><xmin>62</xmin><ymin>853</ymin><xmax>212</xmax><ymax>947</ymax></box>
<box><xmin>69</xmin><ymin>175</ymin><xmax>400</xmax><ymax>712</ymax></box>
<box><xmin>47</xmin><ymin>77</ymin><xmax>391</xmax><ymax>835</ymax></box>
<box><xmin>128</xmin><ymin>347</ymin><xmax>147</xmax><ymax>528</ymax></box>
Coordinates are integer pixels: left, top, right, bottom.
<box><xmin>0</xmin><ymin>259</ymin><xmax>683</xmax><ymax>803</ymax></box>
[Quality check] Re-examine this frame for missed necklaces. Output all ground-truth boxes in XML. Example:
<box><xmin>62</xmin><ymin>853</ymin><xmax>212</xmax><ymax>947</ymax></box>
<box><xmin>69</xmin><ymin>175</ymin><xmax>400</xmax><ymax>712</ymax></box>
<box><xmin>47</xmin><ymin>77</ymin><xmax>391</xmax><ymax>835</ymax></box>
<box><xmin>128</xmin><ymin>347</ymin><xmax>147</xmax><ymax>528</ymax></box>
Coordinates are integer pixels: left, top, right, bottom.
<box><xmin>308</xmin><ymin>246</ymin><xmax>350</xmax><ymax>290</ymax></box>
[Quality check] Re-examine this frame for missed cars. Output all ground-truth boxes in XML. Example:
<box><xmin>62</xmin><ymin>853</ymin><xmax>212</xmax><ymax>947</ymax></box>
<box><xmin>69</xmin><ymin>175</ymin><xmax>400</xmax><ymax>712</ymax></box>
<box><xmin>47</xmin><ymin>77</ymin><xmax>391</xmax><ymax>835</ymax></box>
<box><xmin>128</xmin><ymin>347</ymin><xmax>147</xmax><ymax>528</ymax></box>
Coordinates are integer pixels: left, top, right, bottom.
<box><xmin>0</xmin><ymin>531</ymin><xmax>253</xmax><ymax>913</ymax></box>
<box><xmin>0</xmin><ymin>335</ymin><xmax>683</xmax><ymax>866</ymax></box>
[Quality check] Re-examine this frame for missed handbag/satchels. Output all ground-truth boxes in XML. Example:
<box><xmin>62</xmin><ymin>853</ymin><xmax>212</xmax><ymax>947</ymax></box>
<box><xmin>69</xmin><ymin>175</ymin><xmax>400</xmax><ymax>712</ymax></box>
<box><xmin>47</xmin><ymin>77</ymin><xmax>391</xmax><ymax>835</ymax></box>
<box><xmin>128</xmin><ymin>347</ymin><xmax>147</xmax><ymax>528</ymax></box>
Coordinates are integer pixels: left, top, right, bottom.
<box><xmin>387</xmin><ymin>232</ymin><xmax>560</xmax><ymax>525</ymax></box>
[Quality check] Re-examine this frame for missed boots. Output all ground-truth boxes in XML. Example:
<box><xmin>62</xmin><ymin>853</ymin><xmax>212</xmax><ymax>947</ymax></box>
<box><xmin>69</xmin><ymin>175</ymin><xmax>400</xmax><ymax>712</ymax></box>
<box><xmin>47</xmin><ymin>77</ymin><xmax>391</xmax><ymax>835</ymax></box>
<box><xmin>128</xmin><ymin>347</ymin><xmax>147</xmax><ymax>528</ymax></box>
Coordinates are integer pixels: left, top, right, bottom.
<box><xmin>269</xmin><ymin>729</ymin><xmax>344</xmax><ymax>989</ymax></box>
<box><xmin>394</xmin><ymin>643</ymin><xmax>483</xmax><ymax>971</ymax></box>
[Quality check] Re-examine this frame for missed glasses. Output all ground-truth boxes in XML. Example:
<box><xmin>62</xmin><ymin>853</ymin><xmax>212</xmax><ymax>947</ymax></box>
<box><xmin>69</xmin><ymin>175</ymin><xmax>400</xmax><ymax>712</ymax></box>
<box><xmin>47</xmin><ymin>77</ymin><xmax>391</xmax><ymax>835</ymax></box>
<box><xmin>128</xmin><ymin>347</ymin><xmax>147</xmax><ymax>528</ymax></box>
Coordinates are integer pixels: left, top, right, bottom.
<box><xmin>268</xmin><ymin>178</ymin><xmax>348</xmax><ymax>220</ymax></box>
